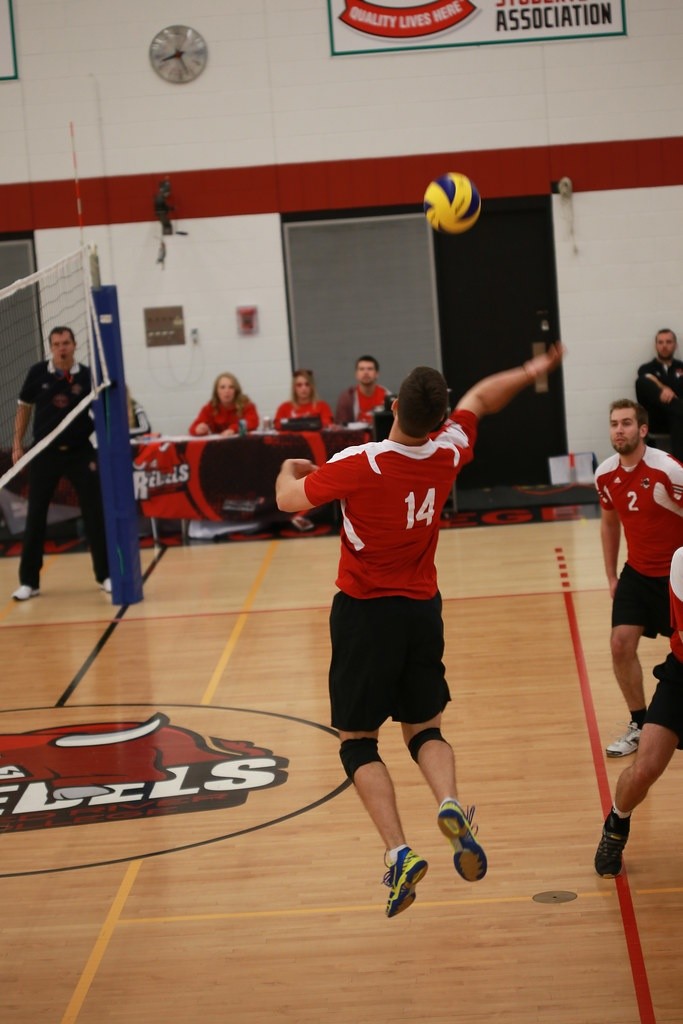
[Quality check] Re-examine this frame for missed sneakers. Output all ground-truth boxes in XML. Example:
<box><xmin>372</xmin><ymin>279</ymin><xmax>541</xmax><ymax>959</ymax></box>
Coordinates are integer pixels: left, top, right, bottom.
<box><xmin>383</xmin><ymin>846</ymin><xmax>429</xmax><ymax>917</ymax></box>
<box><xmin>606</xmin><ymin>720</ymin><xmax>642</xmax><ymax>757</ymax></box>
<box><xmin>98</xmin><ymin>577</ymin><xmax>112</xmax><ymax>593</ymax></box>
<box><xmin>437</xmin><ymin>800</ymin><xmax>488</xmax><ymax>882</ymax></box>
<box><xmin>594</xmin><ymin>806</ymin><xmax>631</xmax><ymax>877</ymax></box>
<box><xmin>10</xmin><ymin>585</ymin><xmax>41</xmax><ymax>601</ymax></box>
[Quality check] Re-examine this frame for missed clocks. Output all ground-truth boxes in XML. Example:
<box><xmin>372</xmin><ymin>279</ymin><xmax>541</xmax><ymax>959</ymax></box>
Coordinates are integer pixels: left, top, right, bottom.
<box><xmin>149</xmin><ymin>25</ymin><xmax>207</xmax><ymax>83</ymax></box>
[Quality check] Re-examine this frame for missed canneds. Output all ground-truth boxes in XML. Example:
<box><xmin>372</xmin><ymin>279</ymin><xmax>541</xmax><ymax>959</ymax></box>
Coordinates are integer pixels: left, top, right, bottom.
<box><xmin>239</xmin><ymin>419</ymin><xmax>246</xmax><ymax>435</ymax></box>
<box><xmin>263</xmin><ymin>416</ymin><xmax>271</xmax><ymax>431</ymax></box>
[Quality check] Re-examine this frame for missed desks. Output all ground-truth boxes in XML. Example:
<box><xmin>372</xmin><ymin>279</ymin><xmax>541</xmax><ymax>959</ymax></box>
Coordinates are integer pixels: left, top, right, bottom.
<box><xmin>133</xmin><ymin>422</ymin><xmax>372</xmax><ymax>550</ymax></box>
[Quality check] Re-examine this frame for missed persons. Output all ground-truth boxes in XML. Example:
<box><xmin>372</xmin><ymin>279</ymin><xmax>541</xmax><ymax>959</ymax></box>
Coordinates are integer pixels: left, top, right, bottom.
<box><xmin>274</xmin><ymin>370</ymin><xmax>336</xmax><ymax>428</ymax></box>
<box><xmin>636</xmin><ymin>329</ymin><xmax>683</xmax><ymax>460</ymax></box>
<box><xmin>127</xmin><ymin>387</ymin><xmax>151</xmax><ymax>438</ymax></box>
<box><xmin>334</xmin><ymin>356</ymin><xmax>392</xmax><ymax>425</ymax></box>
<box><xmin>595</xmin><ymin>399</ymin><xmax>683</xmax><ymax>757</ymax></box>
<box><xmin>275</xmin><ymin>341</ymin><xmax>567</xmax><ymax>916</ymax></box>
<box><xmin>594</xmin><ymin>544</ymin><xmax>683</xmax><ymax>878</ymax></box>
<box><xmin>12</xmin><ymin>327</ymin><xmax>112</xmax><ymax>601</ymax></box>
<box><xmin>188</xmin><ymin>374</ymin><xmax>259</xmax><ymax>436</ymax></box>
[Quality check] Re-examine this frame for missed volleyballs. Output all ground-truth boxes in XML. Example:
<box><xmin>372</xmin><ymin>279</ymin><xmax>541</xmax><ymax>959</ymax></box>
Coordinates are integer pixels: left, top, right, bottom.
<box><xmin>425</xmin><ymin>172</ymin><xmax>482</xmax><ymax>233</ymax></box>
